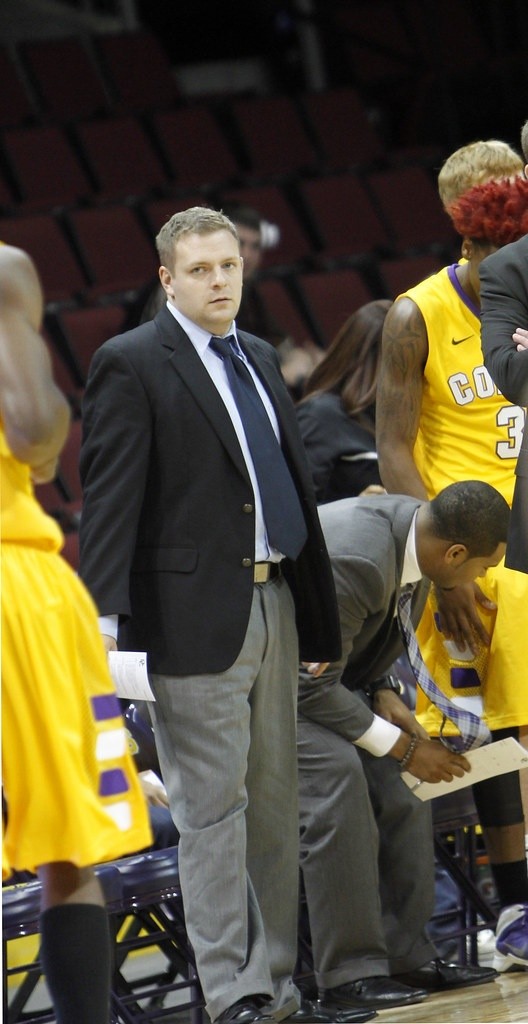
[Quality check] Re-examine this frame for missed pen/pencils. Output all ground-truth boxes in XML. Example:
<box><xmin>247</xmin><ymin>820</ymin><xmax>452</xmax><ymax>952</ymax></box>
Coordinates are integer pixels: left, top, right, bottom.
<box><xmin>411</xmin><ymin>780</ymin><xmax>424</xmax><ymax>791</ymax></box>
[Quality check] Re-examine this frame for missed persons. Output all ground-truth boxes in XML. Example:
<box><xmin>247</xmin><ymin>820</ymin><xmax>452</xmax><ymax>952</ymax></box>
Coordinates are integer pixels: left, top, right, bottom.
<box><xmin>289</xmin><ymin>296</ymin><xmax>427</xmax><ymax>503</ymax></box>
<box><xmin>294</xmin><ymin>481</ymin><xmax>515</xmax><ymax>1012</ymax></box>
<box><xmin>376</xmin><ymin>134</ymin><xmax>528</xmax><ymax>963</ymax></box>
<box><xmin>75</xmin><ymin>205</ymin><xmax>380</xmax><ymax>1023</ymax></box>
<box><xmin>477</xmin><ymin>119</ymin><xmax>528</xmax><ymax>573</ymax></box>
<box><xmin>2</xmin><ymin>238</ymin><xmax>154</xmax><ymax>1024</ymax></box>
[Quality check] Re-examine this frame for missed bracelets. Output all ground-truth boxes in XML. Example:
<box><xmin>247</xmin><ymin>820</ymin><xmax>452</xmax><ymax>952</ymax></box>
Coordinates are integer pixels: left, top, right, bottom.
<box><xmin>397</xmin><ymin>733</ymin><xmax>418</xmax><ymax>768</ymax></box>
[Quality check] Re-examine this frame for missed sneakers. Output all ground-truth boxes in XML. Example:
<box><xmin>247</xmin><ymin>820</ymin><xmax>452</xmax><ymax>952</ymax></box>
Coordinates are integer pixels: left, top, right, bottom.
<box><xmin>493</xmin><ymin>903</ymin><xmax>528</xmax><ymax>973</ymax></box>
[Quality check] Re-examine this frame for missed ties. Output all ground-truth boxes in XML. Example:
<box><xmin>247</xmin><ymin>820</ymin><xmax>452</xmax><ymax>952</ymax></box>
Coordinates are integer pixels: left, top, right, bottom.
<box><xmin>208</xmin><ymin>335</ymin><xmax>309</xmax><ymax>562</ymax></box>
<box><xmin>399</xmin><ymin>583</ymin><xmax>492</xmax><ymax>754</ymax></box>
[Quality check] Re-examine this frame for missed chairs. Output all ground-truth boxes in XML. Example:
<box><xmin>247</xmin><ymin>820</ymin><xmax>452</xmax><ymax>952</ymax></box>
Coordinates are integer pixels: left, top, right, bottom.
<box><xmin>0</xmin><ymin>0</ymin><xmax>528</xmax><ymax>573</ymax></box>
<box><xmin>2</xmin><ymin>697</ymin><xmax>501</xmax><ymax>1024</ymax></box>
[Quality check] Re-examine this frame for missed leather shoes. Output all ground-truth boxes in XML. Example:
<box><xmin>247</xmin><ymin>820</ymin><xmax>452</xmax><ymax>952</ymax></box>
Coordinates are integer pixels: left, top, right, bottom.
<box><xmin>317</xmin><ymin>975</ymin><xmax>429</xmax><ymax>1012</ymax></box>
<box><xmin>389</xmin><ymin>958</ymin><xmax>500</xmax><ymax>994</ymax></box>
<box><xmin>278</xmin><ymin>998</ymin><xmax>379</xmax><ymax>1023</ymax></box>
<box><xmin>218</xmin><ymin>995</ymin><xmax>276</xmax><ymax>1024</ymax></box>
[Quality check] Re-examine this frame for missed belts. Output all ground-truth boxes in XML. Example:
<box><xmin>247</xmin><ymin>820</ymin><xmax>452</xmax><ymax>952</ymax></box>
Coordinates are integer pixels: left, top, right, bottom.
<box><xmin>254</xmin><ymin>561</ymin><xmax>279</xmax><ymax>583</ymax></box>
